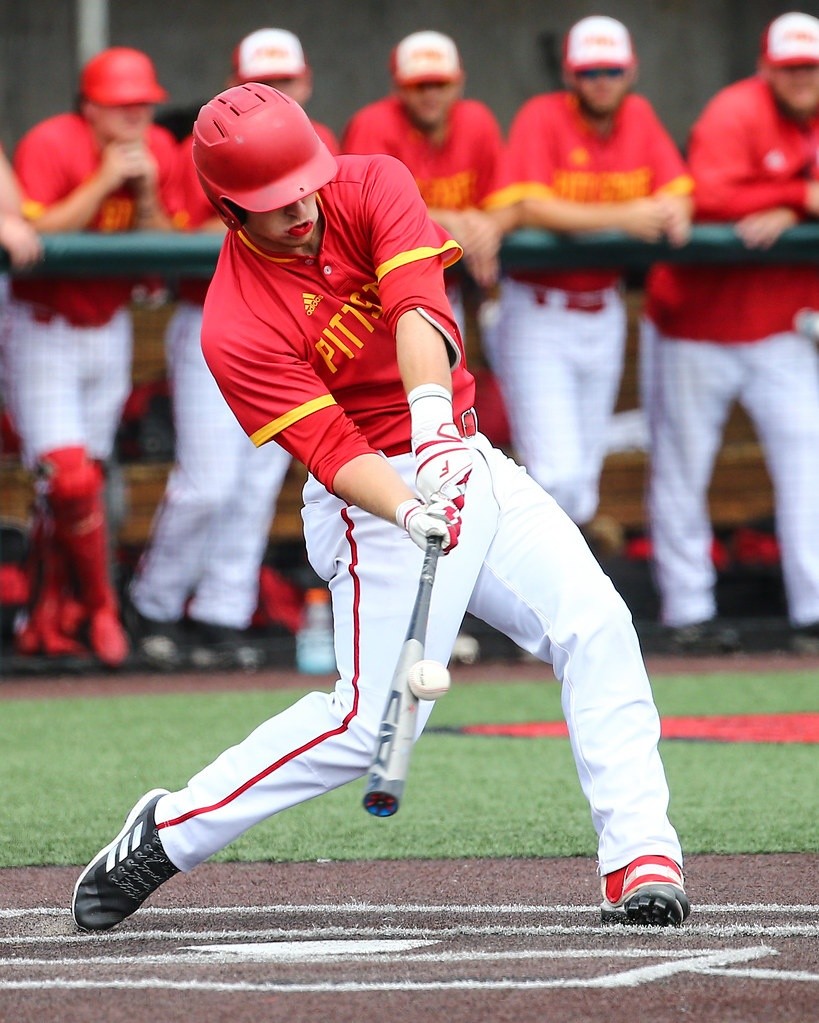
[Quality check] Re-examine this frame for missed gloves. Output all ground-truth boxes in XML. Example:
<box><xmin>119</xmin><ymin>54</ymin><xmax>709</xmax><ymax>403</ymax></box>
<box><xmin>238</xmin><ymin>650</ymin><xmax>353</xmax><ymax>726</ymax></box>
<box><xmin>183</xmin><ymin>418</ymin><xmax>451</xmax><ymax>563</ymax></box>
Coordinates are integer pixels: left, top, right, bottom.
<box><xmin>394</xmin><ymin>498</ymin><xmax>462</xmax><ymax>556</ymax></box>
<box><xmin>407</xmin><ymin>422</ymin><xmax>474</xmax><ymax>509</ymax></box>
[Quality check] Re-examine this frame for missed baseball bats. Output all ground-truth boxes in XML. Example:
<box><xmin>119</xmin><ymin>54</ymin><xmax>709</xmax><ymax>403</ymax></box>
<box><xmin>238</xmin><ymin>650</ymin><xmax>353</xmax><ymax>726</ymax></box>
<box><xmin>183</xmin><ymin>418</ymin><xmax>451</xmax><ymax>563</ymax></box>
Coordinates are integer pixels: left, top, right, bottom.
<box><xmin>361</xmin><ymin>534</ymin><xmax>444</xmax><ymax>818</ymax></box>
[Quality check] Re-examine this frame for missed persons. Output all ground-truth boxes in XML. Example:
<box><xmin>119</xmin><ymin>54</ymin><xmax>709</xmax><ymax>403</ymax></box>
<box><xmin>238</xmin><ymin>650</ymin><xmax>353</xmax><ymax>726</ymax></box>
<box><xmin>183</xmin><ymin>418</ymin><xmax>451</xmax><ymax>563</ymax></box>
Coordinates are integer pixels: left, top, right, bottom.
<box><xmin>125</xmin><ymin>27</ymin><xmax>341</xmax><ymax>671</ymax></box>
<box><xmin>341</xmin><ymin>31</ymin><xmax>525</xmax><ymax>361</ymax></box>
<box><xmin>68</xmin><ymin>82</ymin><xmax>691</xmax><ymax>933</ymax></box>
<box><xmin>0</xmin><ymin>46</ymin><xmax>196</xmax><ymax>667</ymax></box>
<box><xmin>638</xmin><ymin>10</ymin><xmax>819</xmax><ymax>654</ymax></box>
<box><xmin>477</xmin><ymin>17</ymin><xmax>695</xmax><ymax>543</ymax></box>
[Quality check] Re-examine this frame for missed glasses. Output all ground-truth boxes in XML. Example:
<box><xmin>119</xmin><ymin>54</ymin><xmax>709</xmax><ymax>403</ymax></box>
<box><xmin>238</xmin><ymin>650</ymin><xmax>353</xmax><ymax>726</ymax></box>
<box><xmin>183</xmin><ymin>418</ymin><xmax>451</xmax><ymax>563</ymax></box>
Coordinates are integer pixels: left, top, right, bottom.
<box><xmin>577</xmin><ymin>68</ymin><xmax>637</xmax><ymax>79</ymax></box>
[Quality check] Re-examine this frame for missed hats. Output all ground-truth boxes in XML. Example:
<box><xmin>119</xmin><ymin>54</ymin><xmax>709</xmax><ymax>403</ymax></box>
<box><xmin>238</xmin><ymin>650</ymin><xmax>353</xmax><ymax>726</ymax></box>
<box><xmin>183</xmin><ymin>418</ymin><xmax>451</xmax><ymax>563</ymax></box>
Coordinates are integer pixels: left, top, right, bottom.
<box><xmin>759</xmin><ymin>11</ymin><xmax>819</xmax><ymax>68</ymax></box>
<box><xmin>232</xmin><ymin>27</ymin><xmax>308</xmax><ymax>87</ymax></box>
<box><xmin>562</xmin><ymin>16</ymin><xmax>636</xmax><ymax>75</ymax></box>
<box><xmin>390</xmin><ymin>30</ymin><xmax>462</xmax><ymax>86</ymax></box>
<box><xmin>80</xmin><ymin>47</ymin><xmax>164</xmax><ymax>108</ymax></box>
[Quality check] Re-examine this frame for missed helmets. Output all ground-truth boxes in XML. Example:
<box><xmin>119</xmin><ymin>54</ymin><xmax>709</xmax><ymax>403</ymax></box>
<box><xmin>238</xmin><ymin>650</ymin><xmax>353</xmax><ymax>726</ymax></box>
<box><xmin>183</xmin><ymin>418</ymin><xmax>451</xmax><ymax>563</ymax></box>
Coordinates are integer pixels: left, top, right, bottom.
<box><xmin>192</xmin><ymin>82</ymin><xmax>339</xmax><ymax>232</ymax></box>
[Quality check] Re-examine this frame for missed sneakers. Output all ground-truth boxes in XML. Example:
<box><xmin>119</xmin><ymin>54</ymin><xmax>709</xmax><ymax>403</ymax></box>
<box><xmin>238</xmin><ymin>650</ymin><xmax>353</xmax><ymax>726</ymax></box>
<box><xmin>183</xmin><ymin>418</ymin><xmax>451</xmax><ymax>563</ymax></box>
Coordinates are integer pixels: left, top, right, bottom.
<box><xmin>71</xmin><ymin>789</ymin><xmax>180</xmax><ymax>931</ymax></box>
<box><xmin>600</xmin><ymin>855</ymin><xmax>692</xmax><ymax>925</ymax></box>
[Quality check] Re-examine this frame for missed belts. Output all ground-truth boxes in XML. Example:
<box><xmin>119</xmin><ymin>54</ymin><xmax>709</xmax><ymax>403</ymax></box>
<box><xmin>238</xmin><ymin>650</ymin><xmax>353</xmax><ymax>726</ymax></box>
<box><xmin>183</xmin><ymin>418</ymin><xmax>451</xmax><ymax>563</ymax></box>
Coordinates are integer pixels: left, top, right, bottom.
<box><xmin>533</xmin><ymin>287</ymin><xmax>611</xmax><ymax>313</ymax></box>
<box><xmin>31</xmin><ymin>304</ymin><xmax>85</xmax><ymax>327</ymax></box>
<box><xmin>382</xmin><ymin>407</ymin><xmax>479</xmax><ymax>457</ymax></box>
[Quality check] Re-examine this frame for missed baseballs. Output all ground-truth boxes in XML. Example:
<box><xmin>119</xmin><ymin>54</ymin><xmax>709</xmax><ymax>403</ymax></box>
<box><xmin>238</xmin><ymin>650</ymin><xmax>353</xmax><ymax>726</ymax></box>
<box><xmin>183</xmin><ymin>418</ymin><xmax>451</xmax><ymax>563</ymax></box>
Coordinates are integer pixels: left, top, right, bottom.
<box><xmin>406</xmin><ymin>658</ymin><xmax>451</xmax><ymax>702</ymax></box>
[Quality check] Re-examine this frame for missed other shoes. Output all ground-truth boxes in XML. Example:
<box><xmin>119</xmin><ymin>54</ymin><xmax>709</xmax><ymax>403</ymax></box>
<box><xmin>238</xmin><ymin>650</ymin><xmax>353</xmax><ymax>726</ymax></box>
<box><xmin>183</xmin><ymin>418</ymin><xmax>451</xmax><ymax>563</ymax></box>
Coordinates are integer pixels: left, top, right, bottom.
<box><xmin>295</xmin><ymin>603</ymin><xmax>338</xmax><ymax>677</ymax></box>
<box><xmin>645</xmin><ymin>621</ymin><xmax>715</xmax><ymax>657</ymax></box>
<box><xmin>122</xmin><ymin>606</ymin><xmax>182</xmax><ymax>668</ymax></box>
<box><xmin>179</xmin><ymin>615</ymin><xmax>248</xmax><ymax>668</ymax></box>
<box><xmin>90</xmin><ymin>589</ymin><xmax>128</xmax><ymax>664</ymax></box>
<box><xmin>31</xmin><ymin>606</ymin><xmax>81</xmax><ymax>655</ymax></box>
